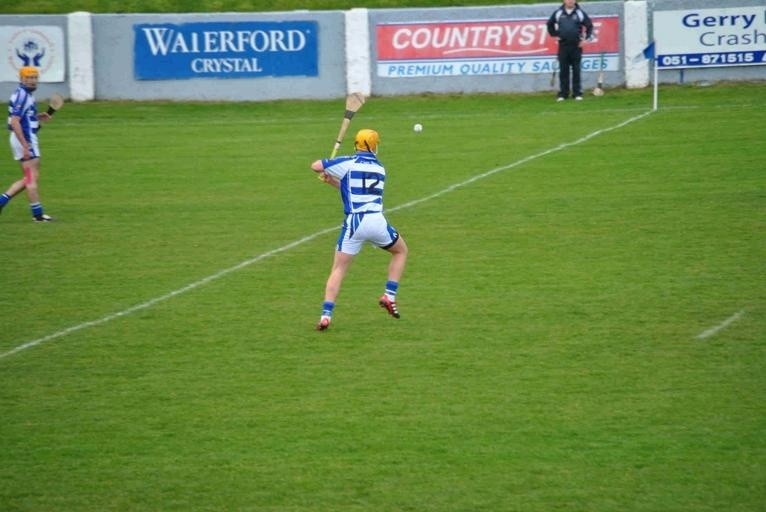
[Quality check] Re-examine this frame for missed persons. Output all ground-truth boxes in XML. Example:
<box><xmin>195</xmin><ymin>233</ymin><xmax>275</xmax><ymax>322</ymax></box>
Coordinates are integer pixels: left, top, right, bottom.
<box><xmin>308</xmin><ymin>128</ymin><xmax>410</xmax><ymax>333</ymax></box>
<box><xmin>546</xmin><ymin>1</ymin><xmax>595</xmax><ymax>101</ymax></box>
<box><xmin>0</xmin><ymin>66</ymin><xmax>55</xmax><ymax>222</ymax></box>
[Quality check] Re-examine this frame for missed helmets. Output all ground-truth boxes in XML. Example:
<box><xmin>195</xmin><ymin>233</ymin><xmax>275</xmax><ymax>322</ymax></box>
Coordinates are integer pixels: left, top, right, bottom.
<box><xmin>19</xmin><ymin>66</ymin><xmax>39</xmax><ymax>78</ymax></box>
<box><xmin>354</xmin><ymin>128</ymin><xmax>380</xmax><ymax>154</ymax></box>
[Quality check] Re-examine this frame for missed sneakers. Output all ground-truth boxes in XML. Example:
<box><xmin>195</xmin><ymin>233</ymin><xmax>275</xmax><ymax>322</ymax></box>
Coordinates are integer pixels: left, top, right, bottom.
<box><xmin>316</xmin><ymin>318</ymin><xmax>329</xmax><ymax>331</ymax></box>
<box><xmin>556</xmin><ymin>96</ymin><xmax>584</xmax><ymax>103</ymax></box>
<box><xmin>379</xmin><ymin>293</ymin><xmax>401</xmax><ymax>319</ymax></box>
<box><xmin>31</xmin><ymin>215</ymin><xmax>54</xmax><ymax>223</ymax></box>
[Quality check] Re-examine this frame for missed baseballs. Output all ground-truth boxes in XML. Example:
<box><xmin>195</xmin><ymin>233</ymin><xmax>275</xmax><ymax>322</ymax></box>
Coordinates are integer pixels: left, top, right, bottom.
<box><xmin>415</xmin><ymin>124</ymin><xmax>422</xmax><ymax>132</ymax></box>
